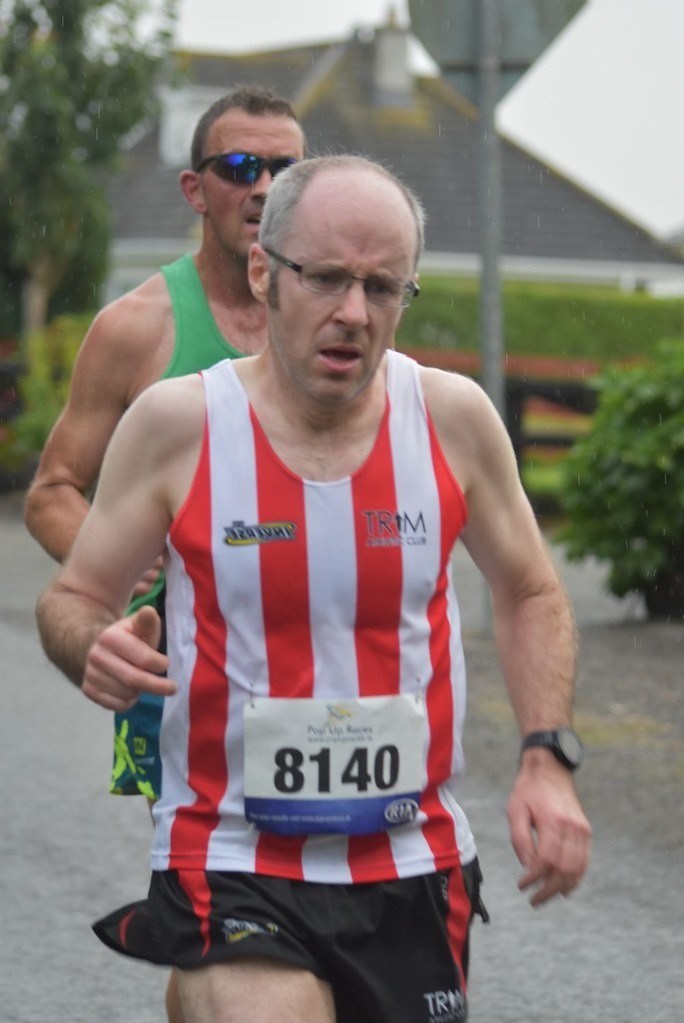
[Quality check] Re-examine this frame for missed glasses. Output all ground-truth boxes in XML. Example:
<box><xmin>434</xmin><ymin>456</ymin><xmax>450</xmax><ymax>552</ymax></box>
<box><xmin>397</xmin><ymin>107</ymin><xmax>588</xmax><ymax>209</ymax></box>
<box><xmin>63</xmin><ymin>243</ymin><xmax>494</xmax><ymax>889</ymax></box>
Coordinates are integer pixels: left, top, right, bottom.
<box><xmin>196</xmin><ymin>153</ymin><xmax>299</xmax><ymax>183</ymax></box>
<box><xmin>264</xmin><ymin>247</ymin><xmax>420</xmax><ymax>308</ymax></box>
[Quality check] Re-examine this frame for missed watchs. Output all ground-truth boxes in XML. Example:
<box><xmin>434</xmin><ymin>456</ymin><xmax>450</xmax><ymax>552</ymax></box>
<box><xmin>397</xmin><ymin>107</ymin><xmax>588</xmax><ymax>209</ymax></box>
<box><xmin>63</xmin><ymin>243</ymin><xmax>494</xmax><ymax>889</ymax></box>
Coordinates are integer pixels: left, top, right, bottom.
<box><xmin>520</xmin><ymin>726</ymin><xmax>584</xmax><ymax>770</ymax></box>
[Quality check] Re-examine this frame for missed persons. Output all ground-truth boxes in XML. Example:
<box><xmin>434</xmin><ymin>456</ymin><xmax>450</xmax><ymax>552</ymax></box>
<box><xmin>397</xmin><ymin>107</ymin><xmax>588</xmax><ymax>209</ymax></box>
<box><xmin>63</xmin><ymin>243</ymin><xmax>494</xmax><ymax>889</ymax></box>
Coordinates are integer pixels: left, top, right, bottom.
<box><xmin>35</xmin><ymin>155</ymin><xmax>593</xmax><ymax>1023</ymax></box>
<box><xmin>23</xmin><ymin>90</ymin><xmax>309</xmax><ymax>829</ymax></box>
